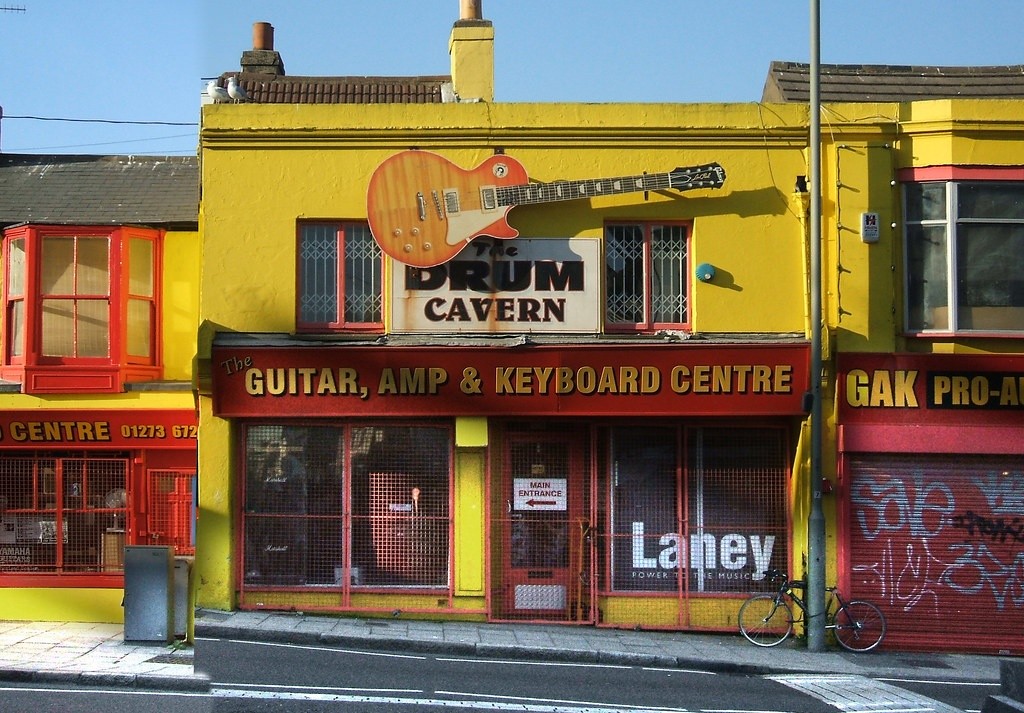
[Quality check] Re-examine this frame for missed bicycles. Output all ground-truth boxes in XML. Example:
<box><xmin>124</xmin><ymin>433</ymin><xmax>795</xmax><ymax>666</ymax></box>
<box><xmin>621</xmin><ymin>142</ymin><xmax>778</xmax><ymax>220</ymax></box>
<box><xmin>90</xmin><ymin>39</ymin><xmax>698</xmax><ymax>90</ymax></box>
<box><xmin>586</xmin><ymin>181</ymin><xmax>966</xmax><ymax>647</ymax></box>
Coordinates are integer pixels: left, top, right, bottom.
<box><xmin>736</xmin><ymin>569</ymin><xmax>889</xmax><ymax>654</ymax></box>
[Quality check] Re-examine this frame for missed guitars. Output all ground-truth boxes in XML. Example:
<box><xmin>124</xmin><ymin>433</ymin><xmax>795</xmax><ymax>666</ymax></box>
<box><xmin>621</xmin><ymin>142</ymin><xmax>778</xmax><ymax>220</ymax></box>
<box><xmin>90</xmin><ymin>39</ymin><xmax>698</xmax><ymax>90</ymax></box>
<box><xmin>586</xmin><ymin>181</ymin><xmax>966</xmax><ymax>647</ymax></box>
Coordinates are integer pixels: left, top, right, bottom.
<box><xmin>366</xmin><ymin>149</ymin><xmax>727</xmax><ymax>269</ymax></box>
